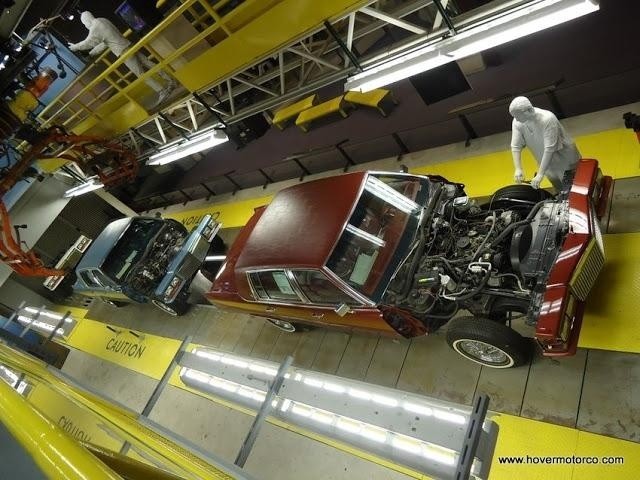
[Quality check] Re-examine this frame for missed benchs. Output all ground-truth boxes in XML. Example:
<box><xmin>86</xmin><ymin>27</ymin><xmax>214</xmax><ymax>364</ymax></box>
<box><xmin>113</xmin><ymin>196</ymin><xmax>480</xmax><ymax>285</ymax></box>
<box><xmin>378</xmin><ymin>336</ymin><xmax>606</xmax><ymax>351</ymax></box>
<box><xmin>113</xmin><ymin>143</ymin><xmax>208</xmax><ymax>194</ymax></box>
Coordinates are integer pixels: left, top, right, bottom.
<box><xmin>343</xmin><ymin>86</ymin><xmax>399</xmax><ymax>117</ymax></box>
<box><xmin>271</xmin><ymin>94</ymin><xmax>321</xmax><ymax>131</ymax></box>
<box><xmin>295</xmin><ymin>94</ymin><xmax>358</xmax><ymax>134</ymax></box>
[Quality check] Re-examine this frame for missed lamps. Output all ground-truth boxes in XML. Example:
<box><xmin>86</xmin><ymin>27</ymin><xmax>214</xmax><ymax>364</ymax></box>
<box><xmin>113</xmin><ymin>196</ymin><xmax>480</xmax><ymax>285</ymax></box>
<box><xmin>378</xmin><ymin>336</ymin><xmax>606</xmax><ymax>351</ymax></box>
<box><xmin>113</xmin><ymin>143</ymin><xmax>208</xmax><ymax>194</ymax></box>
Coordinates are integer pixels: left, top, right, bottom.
<box><xmin>343</xmin><ymin>0</ymin><xmax>601</xmax><ymax>95</ymax></box>
<box><xmin>179</xmin><ymin>343</ymin><xmax>498</xmax><ymax>479</ymax></box>
<box><xmin>18</xmin><ymin>305</ymin><xmax>78</xmax><ymax>343</ymax></box>
<box><xmin>0</xmin><ymin>365</ymin><xmax>34</xmax><ymax>399</ymax></box>
<box><xmin>62</xmin><ymin>174</ymin><xmax>104</xmax><ymax>198</ymax></box>
<box><xmin>143</xmin><ymin>121</ymin><xmax>229</xmax><ymax>166</ymax></box>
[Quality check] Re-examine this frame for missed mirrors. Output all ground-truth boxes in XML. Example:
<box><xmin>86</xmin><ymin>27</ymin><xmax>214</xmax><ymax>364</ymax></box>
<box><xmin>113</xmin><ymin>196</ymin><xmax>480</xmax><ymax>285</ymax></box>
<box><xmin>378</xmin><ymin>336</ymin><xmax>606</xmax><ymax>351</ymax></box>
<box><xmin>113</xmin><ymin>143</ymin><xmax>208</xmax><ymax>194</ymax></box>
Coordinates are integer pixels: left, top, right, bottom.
<box><xmin>2</xmin><ymin>369</ymin><xmax>193</xmax><ymax>477</ymax></box>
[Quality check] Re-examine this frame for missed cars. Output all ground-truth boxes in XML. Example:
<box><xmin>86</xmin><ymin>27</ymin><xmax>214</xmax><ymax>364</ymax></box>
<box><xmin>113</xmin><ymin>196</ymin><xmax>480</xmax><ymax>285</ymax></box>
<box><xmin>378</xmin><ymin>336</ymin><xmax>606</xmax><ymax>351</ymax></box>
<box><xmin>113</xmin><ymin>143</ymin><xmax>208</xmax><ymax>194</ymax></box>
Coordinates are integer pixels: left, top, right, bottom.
<box><xmin>26</xmin><ymin>216</ymin><xmax>92</xmax><ymax>293</ymax></box>
<box><xmin>202</xmin><ymin>160</ymin><xmax>616</xmax><ymax>371</ymax></box>
<box><xmin>69</xmin><ymin>211</ymin><xmax>223</xmax><ymax>317</ymax></box>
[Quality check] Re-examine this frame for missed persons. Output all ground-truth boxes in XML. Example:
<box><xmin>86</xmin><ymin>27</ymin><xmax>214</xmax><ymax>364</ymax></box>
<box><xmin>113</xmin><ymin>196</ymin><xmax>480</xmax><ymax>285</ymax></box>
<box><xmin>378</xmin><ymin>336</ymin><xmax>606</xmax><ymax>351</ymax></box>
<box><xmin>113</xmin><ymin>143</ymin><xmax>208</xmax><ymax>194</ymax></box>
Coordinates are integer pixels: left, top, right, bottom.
<box><xmin>508</xmin><ymin>96</ymin><xmax>582</xmax><ymax>190</ymax></box>
<box><xmin>67</xmin><ymin>11</ymin><xmax>179</xmax><ymax>105</ymax></box>
